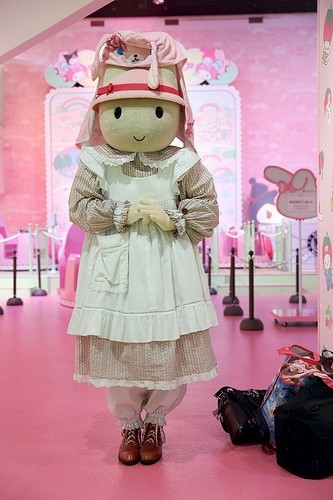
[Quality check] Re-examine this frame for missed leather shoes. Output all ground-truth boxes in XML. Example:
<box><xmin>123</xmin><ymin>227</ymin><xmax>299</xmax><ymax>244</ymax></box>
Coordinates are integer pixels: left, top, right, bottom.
<box><xmin>118</xmin><ymin>426</ymin><xmax>142</xmax><ymax>467</ymax></box>
<box><xmin>138</xmin><ymin>422</ymin><xmax>165</xmax><ymax>465</ymax></box>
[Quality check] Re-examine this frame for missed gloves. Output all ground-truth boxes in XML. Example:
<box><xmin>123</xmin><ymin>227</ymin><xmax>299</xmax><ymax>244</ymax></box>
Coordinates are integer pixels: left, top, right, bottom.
<box><xmin>123</xmin><ymin>198</ymin><xmax>144</xmax><ymax>227</ymax></box>
<box><xmin>138</xmin><ymin>200</ymin><xmax>177</xmax><ymax>233</ymax></box>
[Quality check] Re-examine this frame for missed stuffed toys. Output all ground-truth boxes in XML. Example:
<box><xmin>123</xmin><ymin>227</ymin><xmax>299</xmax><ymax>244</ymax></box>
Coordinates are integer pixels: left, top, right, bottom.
<box><xmin>64</xmin><ymin>29</ymin><xmax>219</xmax><ymax>466</ymax></box>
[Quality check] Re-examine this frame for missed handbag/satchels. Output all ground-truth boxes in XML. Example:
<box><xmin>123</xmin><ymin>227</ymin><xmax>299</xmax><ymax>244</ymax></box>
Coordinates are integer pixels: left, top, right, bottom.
<box><xmin>214</xmin><ymin>385</ymin><xmax>271</xmax><ymax>445</ymax></box>
<box><xmin>259</xmin><ymin>343</ymin><xmax>333</xmax><ymax>449</ymax></box>
<box><xmin>273</xmin><ymin>375</ymin><xmax>333</xmax><ymax>479</ymax></box>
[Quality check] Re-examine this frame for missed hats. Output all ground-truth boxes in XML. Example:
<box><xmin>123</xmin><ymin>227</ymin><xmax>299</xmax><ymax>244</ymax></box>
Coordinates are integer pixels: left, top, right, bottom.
<box><xmin>88</xmin><ymin>30</ymin><xmax>189</xmax><ymax>109</ymax></box>
